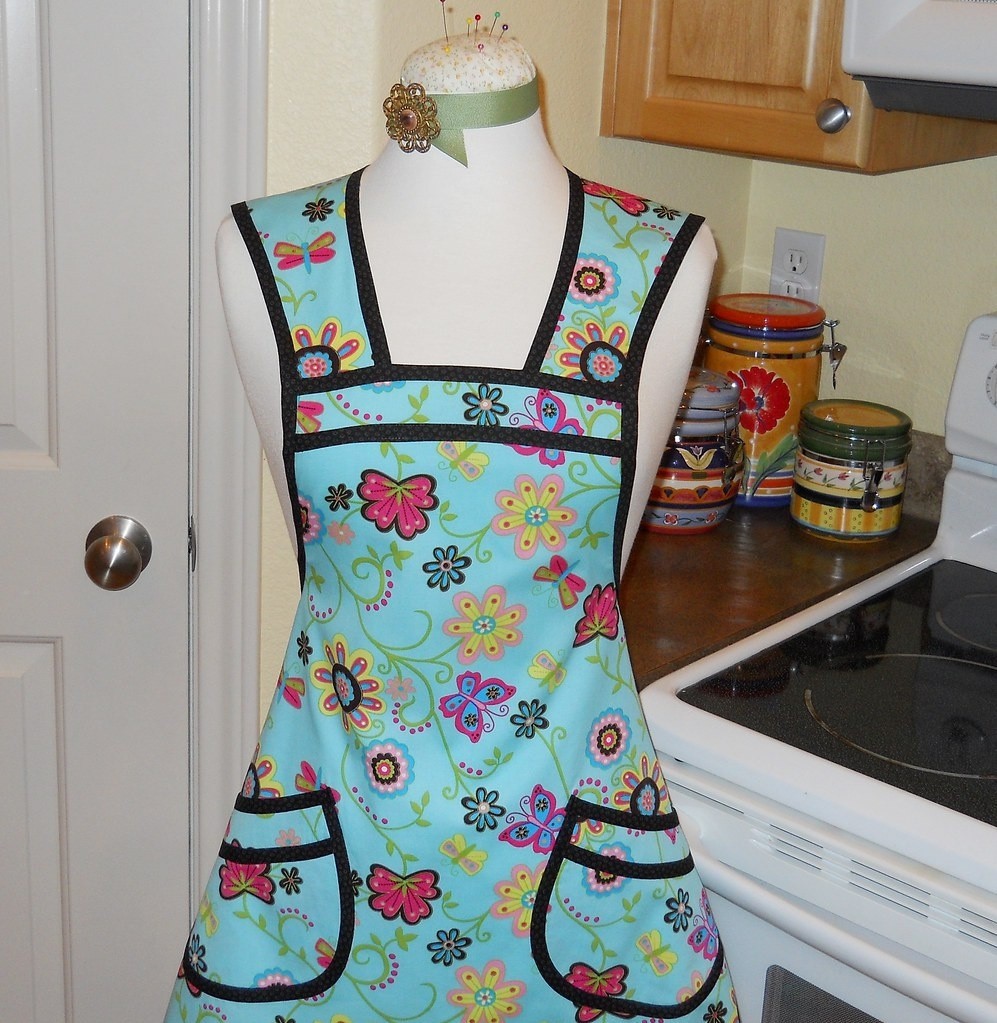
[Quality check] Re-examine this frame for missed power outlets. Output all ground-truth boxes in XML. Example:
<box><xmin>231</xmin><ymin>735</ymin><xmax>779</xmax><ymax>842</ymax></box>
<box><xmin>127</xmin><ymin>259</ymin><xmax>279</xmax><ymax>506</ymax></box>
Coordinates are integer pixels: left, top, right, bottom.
<box><xmin>768</xmin><ymin>227</ymin><xmax>825</xmax><ymax>308</ymax></box>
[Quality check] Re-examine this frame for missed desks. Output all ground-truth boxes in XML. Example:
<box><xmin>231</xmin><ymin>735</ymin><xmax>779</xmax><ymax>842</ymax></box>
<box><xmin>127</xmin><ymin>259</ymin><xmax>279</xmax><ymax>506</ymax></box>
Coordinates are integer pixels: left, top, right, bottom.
<box><xmin>617</xmin><ymin>503</ymin><xmax>939</xmax><ymax>691</ymax></box>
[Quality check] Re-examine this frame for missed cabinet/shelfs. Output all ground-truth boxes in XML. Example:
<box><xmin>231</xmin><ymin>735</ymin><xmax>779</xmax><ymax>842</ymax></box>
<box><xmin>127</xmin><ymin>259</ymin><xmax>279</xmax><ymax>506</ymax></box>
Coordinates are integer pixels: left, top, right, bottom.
<box><xmin>599</xmin><ymin>0</ymin><xmax>997</xmax><ymax>177</ymax></box>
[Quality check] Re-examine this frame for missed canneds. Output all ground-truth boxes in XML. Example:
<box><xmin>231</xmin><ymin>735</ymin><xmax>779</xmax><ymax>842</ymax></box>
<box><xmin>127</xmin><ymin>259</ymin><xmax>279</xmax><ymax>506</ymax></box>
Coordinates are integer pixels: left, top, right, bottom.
<box><xmin>641</xmin><ymin>294</ymin><xmax>912</xmax><ymax>544</ymax></box>
